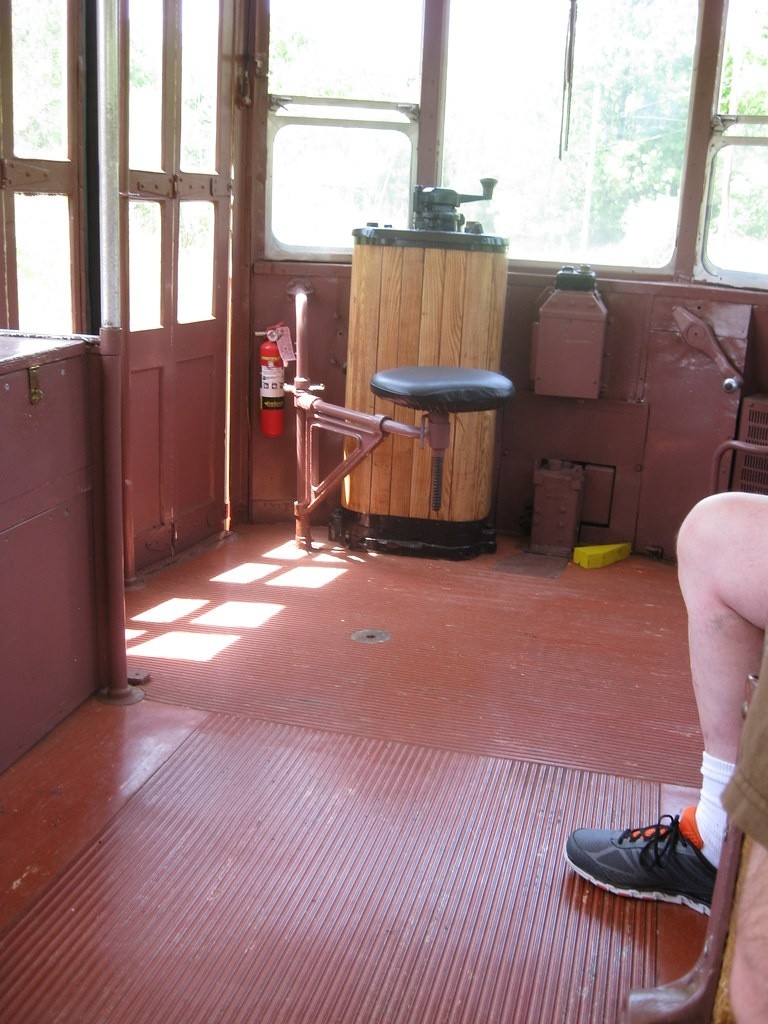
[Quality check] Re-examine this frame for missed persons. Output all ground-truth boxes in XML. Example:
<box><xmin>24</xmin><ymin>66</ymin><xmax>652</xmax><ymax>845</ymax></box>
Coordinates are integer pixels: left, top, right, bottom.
<box><xmin>563</xmin><ymin>492</ymin><xmax>768</xmax><ymax>916</ymax></box>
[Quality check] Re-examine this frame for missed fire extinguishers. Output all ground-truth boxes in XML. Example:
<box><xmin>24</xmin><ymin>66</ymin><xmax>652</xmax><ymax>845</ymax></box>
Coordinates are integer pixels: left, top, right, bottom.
<box><xmin>259</xmin><ymin>321</ymin><xmax>286</xmax><ymax>438</ymax></box>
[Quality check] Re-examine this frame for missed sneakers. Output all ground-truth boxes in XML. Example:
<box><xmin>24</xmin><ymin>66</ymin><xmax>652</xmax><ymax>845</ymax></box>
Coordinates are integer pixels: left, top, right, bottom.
<box><xmin>565</xmin><ymin>806</ymin><xmax>717</xmax><ymax>916</ymax></box>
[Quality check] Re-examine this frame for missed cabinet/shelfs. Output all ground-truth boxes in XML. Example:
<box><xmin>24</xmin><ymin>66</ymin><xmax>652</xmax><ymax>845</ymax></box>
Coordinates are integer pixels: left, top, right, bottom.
<box><xmin>0</xmin><ymin>334</ymin><xmax>102</xmax><ymax>775</ymax></box>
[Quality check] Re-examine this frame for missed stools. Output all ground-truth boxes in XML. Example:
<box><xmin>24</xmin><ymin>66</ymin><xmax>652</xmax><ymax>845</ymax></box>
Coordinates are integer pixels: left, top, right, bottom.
<box><xmin>371</xmin><ymin>367</ymin><xmax>516</xmax><ymax>512</ymax></box>
